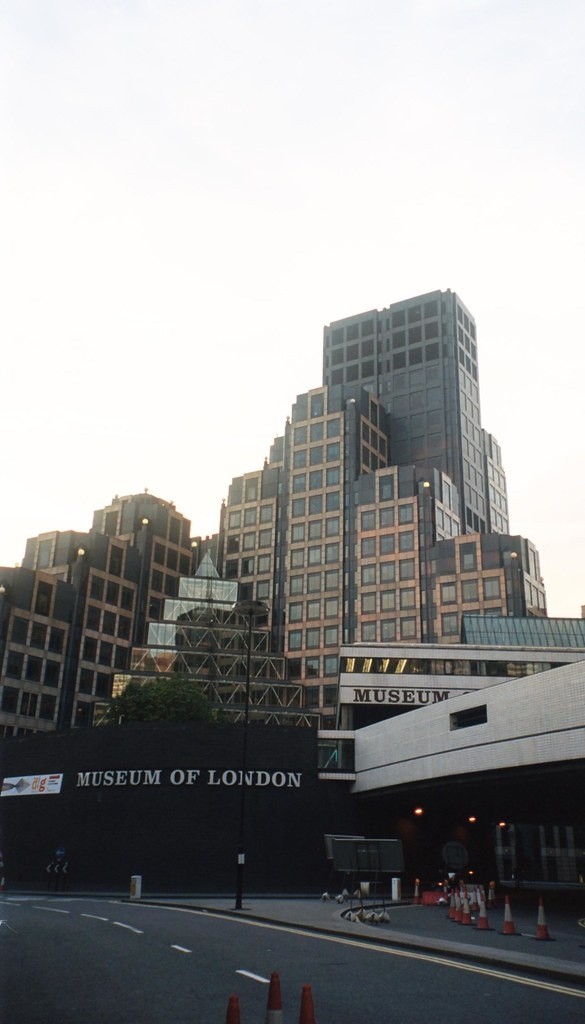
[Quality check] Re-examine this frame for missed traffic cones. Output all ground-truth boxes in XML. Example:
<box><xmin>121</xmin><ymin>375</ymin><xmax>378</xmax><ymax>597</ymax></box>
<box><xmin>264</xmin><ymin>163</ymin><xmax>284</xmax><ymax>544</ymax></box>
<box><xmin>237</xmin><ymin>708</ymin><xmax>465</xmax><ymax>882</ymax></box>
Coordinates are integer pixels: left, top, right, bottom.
<box><xmin>530</xmin><ymin>896</ymin><xmax>556</xmax><ymax>941</ymax></box>
<box><xmin>498</xmin><ymin>894</ymin><xmax>522</xmax><ymax>936</ymax></box>
<box><xmin>225</xmin><ymin>993</ymin><xmax>241</xmax><ymax>1024</ymax></box>
<box><xmin>265</xmin><ymin>972</ymin><xmax>285</xmax><ymax>1024</ymax></box>
<box><xmin>439</xmin><ymin>879</ymin><xmax>496</xmax><ymax>926</ymax></box>
<box><xmin>472</xmin><ymin>894</ymin><xmax>495</xmax><ymax>931</ymax></box>
<box><xmin>298</xmin><ymin>984</ymin><xmax>316</xmax><ymax>1024</ymax></box>
<box><xmin>411</xmin><ymin>879</ymin><xmax>422</xmax><ymax>905</ymax></box>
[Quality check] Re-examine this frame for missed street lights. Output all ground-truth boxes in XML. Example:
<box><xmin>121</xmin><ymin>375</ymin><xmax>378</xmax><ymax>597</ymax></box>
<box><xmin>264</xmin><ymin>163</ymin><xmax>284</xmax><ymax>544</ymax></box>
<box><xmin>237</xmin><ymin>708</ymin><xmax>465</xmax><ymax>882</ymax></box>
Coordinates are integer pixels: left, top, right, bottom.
<box><xmin>232</xmin><ymin>599</ymin><xmax>271</xmax><ymax>909</ymax></box>
